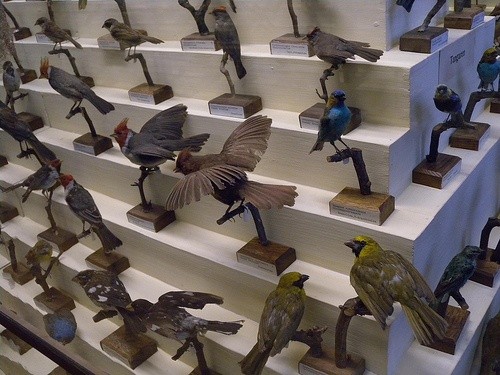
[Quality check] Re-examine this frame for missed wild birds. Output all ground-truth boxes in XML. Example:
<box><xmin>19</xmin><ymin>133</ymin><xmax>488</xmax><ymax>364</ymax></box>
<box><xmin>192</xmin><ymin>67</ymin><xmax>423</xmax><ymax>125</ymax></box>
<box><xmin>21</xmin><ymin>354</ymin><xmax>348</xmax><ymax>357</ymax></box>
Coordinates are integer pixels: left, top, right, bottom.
<box><xmin>434</xmin><ymin>243</ymin><xmax>486</xmax><ymax>313</ymax></box>
<box><xmin>0</xmin><ymin>100</ymin><xmax>56</xmax><ymax>165</ymax></box>
<box><xmin>101</xmin><ymin>17</ymin><xmax>165</xmax><ymax>62</ymax></box>
<box><xmin>236</xmin><ymin>272</ymin><xmax>323</xmax><ymax>375</ymax></box>
<box><xmin>33</xmin><ymin>16</ymin><xmax>84</xmax><ymax>52</ymax></box>
<box><xmin>3</xmin><ymin>61</ymin><xmax>25</xmax><ymax>106</ymax></box>
<box><xmin>302</xmin><ymin>26</ymin><xmax>384</xmax><ymax>80</ymax></box>
<box><xmin>123</xmin><ymin>290</ymin><xmax>244</xmax><ymax>353</ymax></box>
<box><xmin>164</xmin><ymin>113</ymin><xmax>297</xmax><ymax>225</ymax></box>
<box><xmin>433</xmin><ymin>84</ymin><xmax>465</xmax><ymax>125</ymax></box>
<box><xmin>344</xmin><ymin>235</ymin><xmax>451</xmax><ymax>346</ymax></box>
<box><xmin>71</xmin><ymin>269</ymin><xmax>132</xmax><ymax>311</ymax></box>
<box><xmin>476</xmin><ymin>48</ymin><xmax>500</xmax><ymax>91</ymax></box>
<box><xmin>308</xmin><ymin>90</ymin><xmax>353</xmax><ymax>158</ymax></box>
<box><xmin>3</xmin><ymin>159</ymin><xmax>124</xmax><ymax>255</ymax></box>
<box><xmin>208</xmin><ymin>6</ymin><xmax>248</xmax><ymax>80</ymax></box>
<box><xmin>110</xmin><ymin>104</ymin><xmax>211</xmax><ymax>183</ymax></box>
<box><xmin>37</xmin><ymin>56</ymin><xmax>115</xmax><ymax>116</ymax></box>
